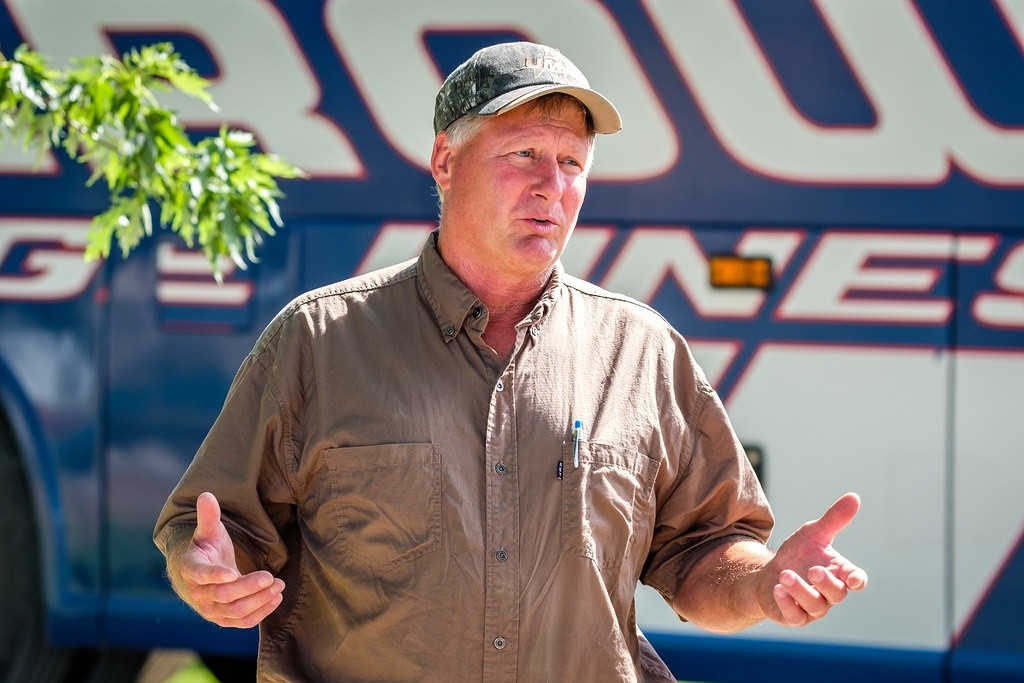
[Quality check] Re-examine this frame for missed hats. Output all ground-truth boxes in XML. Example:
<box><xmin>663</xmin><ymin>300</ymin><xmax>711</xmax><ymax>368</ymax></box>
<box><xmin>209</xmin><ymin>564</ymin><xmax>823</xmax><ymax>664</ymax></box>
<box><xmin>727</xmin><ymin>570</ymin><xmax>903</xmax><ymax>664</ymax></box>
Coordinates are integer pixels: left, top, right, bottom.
<box><xmin>433</xmin><ymin>40</ymin><xmax>622</xmax><ymax>136</ymax></box>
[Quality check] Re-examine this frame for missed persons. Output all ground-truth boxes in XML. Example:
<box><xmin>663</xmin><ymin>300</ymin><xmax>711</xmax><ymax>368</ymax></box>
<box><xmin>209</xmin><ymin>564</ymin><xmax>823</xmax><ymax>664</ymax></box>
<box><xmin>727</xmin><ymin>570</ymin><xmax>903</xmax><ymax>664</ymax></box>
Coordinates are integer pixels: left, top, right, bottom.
<box><xmin>149</xmin><ymin>41</ymin><xmax>869</xmax><ymax>683</ymax></box>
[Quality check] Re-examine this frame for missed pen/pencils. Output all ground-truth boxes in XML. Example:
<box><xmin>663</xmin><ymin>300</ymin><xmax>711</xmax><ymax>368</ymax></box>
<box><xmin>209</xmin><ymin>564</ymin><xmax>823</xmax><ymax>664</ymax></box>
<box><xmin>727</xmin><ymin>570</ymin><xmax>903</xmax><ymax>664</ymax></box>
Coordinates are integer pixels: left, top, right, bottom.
<box><xmin>571</xmin><ymin>420</ymin><xmax>584</xmax><ymax>469</ymax></box>
